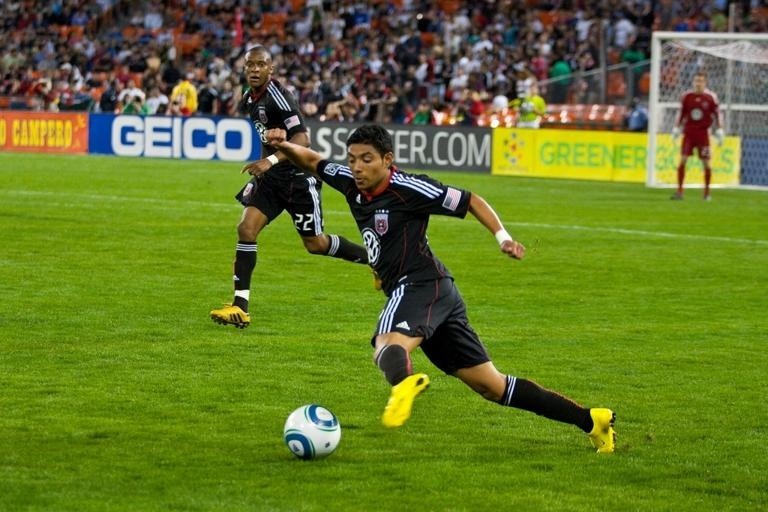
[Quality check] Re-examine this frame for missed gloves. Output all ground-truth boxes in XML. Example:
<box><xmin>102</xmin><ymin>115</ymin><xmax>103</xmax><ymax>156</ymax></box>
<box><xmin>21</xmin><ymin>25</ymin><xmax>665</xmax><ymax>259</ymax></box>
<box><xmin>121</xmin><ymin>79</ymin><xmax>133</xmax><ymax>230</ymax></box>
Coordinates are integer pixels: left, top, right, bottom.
<box><xmin>714</xmin><ymin>128</ymin><xmax>725</xmax><ymax>147</ymax></box>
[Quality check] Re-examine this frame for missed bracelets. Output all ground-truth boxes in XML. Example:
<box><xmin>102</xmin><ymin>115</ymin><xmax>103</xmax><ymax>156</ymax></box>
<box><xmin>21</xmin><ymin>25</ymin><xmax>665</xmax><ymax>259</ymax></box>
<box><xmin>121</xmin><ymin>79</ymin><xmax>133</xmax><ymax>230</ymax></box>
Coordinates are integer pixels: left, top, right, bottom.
<box><xmin>494</xmin><ymin>229</ymin><xmax>514</xmax><ymax>245</ymax></box>
<box><xmin>265</xmin><ymin>155</ymin><xmax>280</xmax><ymax>165</ymax></box>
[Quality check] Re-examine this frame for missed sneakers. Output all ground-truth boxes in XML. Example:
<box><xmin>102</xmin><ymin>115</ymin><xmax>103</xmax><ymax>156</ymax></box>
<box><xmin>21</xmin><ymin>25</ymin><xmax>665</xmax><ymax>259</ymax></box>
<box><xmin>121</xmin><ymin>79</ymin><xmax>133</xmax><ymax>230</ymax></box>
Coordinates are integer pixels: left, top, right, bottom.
<box><xmin>210</xmin><ymin>302</ymin><xmax>251</xmax><ymax>329</ymax></box>
<box><xmin>371</xmin><ymin>269</ymin><xmax>383</xmax><ymax>290</ymax></box>
<box><xmin>671</xmin><ymin>192</ymin><xmax>684</xmax><ymax>200</ymax></box>
<box><xmin>382</xmin><ymin>373</ymin><xmax>431</xmax><ymax>427</ymax></box>
<box><xmin>586</xmin><ymin>408</ymin><xmax>617</xmax><ymax>454</ymax></box>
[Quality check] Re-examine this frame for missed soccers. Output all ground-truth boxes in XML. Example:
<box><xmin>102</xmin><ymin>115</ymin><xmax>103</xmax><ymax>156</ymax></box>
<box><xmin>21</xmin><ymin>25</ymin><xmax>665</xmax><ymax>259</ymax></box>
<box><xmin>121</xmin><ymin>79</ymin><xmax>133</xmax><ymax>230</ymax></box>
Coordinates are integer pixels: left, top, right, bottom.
<box><xmin>284</xmin><ymin>405</ymin><xmax>341</xmax><ymax>459</ymax></box>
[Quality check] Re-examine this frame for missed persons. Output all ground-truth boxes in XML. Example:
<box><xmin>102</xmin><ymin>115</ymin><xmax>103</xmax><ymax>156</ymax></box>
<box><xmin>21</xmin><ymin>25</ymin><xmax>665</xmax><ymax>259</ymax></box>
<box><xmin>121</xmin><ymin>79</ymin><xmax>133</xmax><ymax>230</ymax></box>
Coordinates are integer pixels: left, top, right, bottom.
<box><xmin>264</xmin><ymin>123</ymin><xmax>618</xmax><ymax>455</ymax></box>
<box><xmin>669</xmin><ymin>72</ymin><xmax>725</xmax><ymax>201</ymax></box>
<box><xmin>0</xmin><ymin>1</ymin><xmax>767</xmax><ymax>134</ymax></box>
<box><xmin>209</xmin><ymin>45</ymin><xmax>383</xmax><ymax>330</ymax></box>
